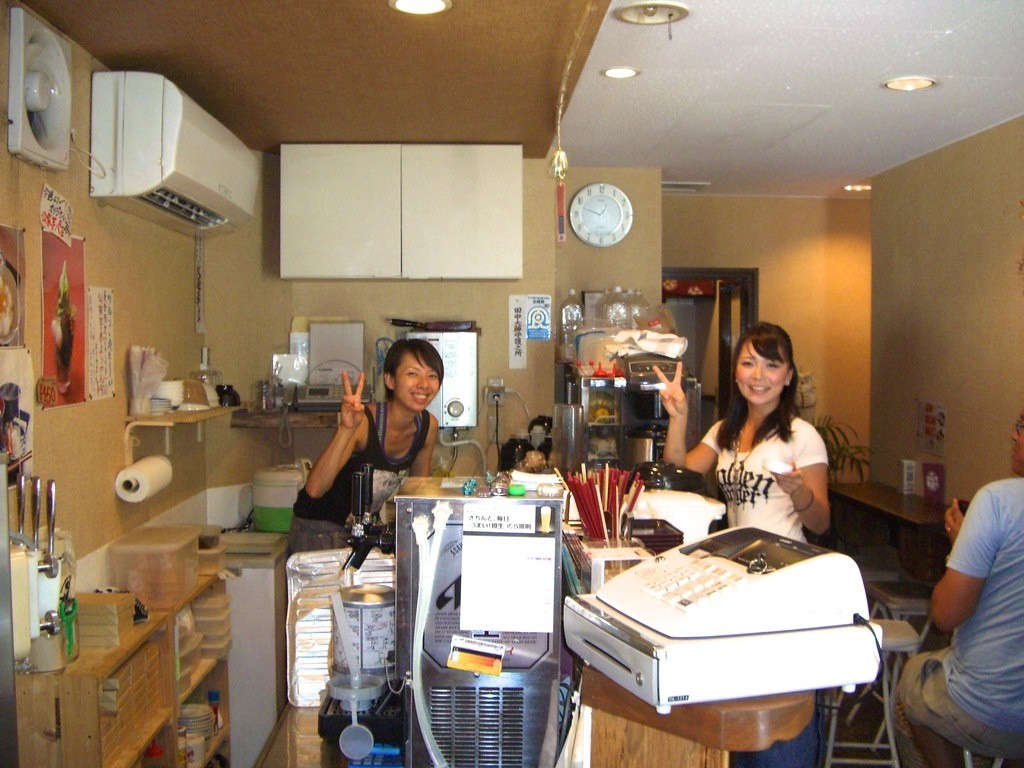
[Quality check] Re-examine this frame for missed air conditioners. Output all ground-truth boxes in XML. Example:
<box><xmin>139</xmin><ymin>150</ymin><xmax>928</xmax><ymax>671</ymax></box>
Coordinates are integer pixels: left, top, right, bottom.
<box><xmin>88</xmin><ymin>72</ymin><xmax>259</xmax><ymax>239</ymax></box>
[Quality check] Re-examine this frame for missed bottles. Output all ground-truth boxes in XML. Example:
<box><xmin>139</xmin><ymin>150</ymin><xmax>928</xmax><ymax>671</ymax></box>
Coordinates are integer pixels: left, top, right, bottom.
<box><xmin>596</xmin><ymin>286</ymin><xmax>648</xmax><ymax>330</ymax></box>
<box><xmin>146</xmin><ymin>739</ymin><xmax>168</xmax><ymax>768</ymax></box>
<box><xmin>560</xmin><ymin>289</ymin><xmax>585</xmax><ymax>359</ymax></box>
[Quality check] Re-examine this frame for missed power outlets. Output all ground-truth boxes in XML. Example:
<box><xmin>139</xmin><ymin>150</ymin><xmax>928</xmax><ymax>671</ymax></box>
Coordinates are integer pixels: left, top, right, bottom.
<box><xmin>487</xmin><ymin>391</ymin><xmax>503</xmax><ymax>406</ymax></box>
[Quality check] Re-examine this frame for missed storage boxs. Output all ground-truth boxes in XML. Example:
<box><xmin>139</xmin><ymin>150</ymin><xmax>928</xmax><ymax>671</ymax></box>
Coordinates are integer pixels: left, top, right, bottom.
<box><xmin>197</xmin><ymin>542</ymin><xmax>227</xmax><ymax>575</ymax></box>
<box><xmin>109</xmin><ymin>523</ymin><xmax>201</xmax><ymax>610</ymax></box>
<box><xmin>75</xmin><ymin>591</ymin><xmax>136</xmax><ymax>648</ymax></box>
<box><xmin>174</xmin><ymin>592</ymin><xmax>232</xmax><ymax>695</ymax></box>
<box><xmin>197</xmin><ymin>523</ymin><xmax>220</xmax><ymax>549</ymax></box>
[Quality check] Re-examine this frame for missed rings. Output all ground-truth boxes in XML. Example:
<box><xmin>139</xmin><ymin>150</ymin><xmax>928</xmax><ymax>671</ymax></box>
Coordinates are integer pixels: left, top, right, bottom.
<box><xmin>947</xmin><ymin>526</ymin><xmax>951</xmax><ymax>535</ymax></box>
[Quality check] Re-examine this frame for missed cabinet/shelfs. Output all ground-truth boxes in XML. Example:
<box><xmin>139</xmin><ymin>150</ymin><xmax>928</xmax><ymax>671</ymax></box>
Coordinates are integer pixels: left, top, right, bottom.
<box><xmin>17</xmin><ymin>572</ymin><xmax>232</xmax><ymax>768</ymax></box>
<box><xmin>279</xmin><ymin>141</ymin><xmax>524</xmax><ymax>283</ymax></box>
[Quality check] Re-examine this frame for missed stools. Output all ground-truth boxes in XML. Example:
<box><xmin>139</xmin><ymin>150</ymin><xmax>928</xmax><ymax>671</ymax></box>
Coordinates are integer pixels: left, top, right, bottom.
<box><xmin>822</xmin><ymin>619</ymin><xmax>921</xmax><ymax>768</ymax></box>
<box><xmin>846</xmin><ymin>579</ymin><xmax>935</xmax><ymax>751</ymax></box>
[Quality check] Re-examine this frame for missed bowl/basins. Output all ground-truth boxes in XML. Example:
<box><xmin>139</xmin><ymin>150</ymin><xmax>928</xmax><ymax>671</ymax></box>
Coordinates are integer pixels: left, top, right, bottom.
<box><xmin>150</xmin><ymin>380</ymin><xmax>240</xmax><ymax>415</ymax></box>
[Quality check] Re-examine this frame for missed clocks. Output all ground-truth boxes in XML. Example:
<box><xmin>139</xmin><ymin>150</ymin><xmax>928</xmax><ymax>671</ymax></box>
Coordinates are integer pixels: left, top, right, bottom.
<box><xmin>569</xmin><ymin>182</ymin><xmax>633</xmax><ymax>247</ymax></box>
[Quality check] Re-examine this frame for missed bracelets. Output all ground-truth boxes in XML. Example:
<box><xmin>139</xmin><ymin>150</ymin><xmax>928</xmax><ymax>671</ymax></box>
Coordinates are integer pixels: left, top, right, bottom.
<box><xmin>793</xmin><ymin>490</ymin><xmax>816</xmax><ymax>515</ymax></box>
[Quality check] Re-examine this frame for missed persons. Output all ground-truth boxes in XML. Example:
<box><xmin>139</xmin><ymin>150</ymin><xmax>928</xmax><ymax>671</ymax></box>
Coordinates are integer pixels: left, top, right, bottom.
<box><xmin>284</xmin><ymin>338</ymin><xmax>445</xmax><ymax>562</ymax></box>
<box><xmin>892</xmin><ymin>406</ymin><xmax>1024</xmax><ymax>768</ymax></box>
<box><xmin>651</xmin><ymin>321</ymin><xmax>831</xmax><ymax>545</ymax></box>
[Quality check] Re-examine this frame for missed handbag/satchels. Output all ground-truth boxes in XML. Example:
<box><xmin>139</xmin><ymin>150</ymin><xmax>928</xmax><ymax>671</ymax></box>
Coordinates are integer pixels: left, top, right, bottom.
<box><xmin>793</xmin><ymin>367</ymin><xmax>816</xmax><ymax>408</ymax></box>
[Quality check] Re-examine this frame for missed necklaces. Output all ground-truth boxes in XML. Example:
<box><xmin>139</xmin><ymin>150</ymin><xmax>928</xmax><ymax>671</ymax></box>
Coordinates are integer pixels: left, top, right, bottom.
<box><xmin>720</xmin><ymin>423</ymin><xmax>773</xmax><ymax>484</ymax></box>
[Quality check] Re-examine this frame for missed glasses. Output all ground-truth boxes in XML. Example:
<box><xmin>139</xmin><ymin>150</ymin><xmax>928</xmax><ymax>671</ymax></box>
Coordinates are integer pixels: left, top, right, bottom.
<box><xmin>1015</xmin><ymin>421</ymin><xmax>1024</xmax><ymax>435</ymax></box>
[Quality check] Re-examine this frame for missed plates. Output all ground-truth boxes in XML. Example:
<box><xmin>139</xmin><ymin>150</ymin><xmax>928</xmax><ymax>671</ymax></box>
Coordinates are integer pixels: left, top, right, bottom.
<box><xmin>178</xmin><ymin>703</ymin><xmax>215</xmax><ymax>738</ymax></box>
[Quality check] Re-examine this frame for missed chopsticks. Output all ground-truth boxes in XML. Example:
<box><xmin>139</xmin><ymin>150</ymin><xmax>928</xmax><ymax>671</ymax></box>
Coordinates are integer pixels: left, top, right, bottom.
<box><xmin>554</xmin><ymin>462</ymin><xmax>645</xmax><ymax>571</ymax></box>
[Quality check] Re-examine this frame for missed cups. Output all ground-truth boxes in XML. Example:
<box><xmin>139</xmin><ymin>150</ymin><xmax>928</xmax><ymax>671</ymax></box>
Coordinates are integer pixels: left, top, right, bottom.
<box><xmin>958</xmin><ymin>499</ymin><xmax>970</xmax><ymax>516</ymax></box>
<box><xmin>129</xmin><ymin>399</ymin><xmax>150</xmax><ymax>416</ymax></box>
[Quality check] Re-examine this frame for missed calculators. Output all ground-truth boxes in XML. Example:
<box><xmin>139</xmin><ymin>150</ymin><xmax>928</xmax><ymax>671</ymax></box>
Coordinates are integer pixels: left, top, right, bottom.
<box><xmin>347</xmin><ymin>740</ymin><xmax>403</xmax><ymax>768</ymax></box>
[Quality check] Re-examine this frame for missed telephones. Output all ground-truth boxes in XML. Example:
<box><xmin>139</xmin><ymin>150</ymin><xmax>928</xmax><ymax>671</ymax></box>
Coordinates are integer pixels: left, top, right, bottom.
<box><xmin>283</xmin><ymin>382</ymin><xmax>371</xmax><ymax>411</ymax></box>
